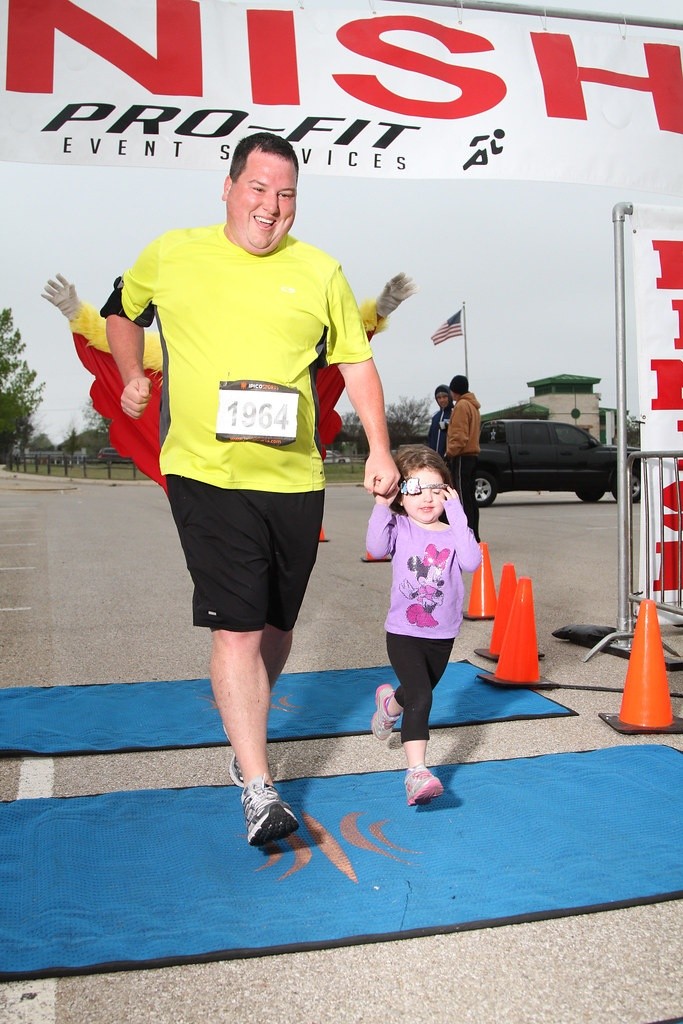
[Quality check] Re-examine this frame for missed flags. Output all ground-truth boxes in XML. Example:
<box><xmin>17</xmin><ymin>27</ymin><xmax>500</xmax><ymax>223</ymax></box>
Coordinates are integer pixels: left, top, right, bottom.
<box><xmin>430</xmin><ymin>308</ymin><xmax>462</xmax><ymax>345</ymax></box>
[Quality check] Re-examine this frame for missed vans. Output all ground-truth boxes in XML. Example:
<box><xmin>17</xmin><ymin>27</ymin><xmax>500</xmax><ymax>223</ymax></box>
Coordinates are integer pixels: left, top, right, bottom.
<box><xmin>98</xmin><ymin>447</ymin><xmax>132</xmax><ymax>464</ymax></box>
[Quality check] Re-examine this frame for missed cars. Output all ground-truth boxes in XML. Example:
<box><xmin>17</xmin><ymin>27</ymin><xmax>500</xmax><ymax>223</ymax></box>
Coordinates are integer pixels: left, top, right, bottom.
<box><xmin>323</xmin><ymin>451</ymin><xmax>351</xmax><ymax>464</ymax></box>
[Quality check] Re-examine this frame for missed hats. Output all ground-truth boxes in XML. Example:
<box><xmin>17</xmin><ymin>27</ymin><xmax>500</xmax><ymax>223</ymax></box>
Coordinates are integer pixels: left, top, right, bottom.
<box><xmin>435</xmin><ymin>387</ymin><xmax>449</xmax><ymax>397</ymax></box>
<box><xmin>449</xmin><ymin>375</ymin><xmax>468</xmax><ymax>397</ymax></box>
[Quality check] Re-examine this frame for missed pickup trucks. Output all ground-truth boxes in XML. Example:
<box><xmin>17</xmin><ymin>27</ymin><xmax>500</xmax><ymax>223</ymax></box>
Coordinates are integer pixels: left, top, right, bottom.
<box><xmin>470</xmin><ymin>417</ymin><xmax>641</xmax><ymax>506</ymax></box>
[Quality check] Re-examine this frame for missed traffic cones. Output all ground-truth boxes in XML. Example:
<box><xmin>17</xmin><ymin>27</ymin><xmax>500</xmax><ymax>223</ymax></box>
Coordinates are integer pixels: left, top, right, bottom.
<box><xmin>476</xmin><ymin>575</ymin><xmax>560</xmax><ymax>689</ymax></box>
<box><xmin>359</xmin><ymin>549</ymin><xmax>392</xmax><ymax>562</ymax></box>
<box><xmin>319</xmin><ymin>528</ymin><xmax>328</xmax><ymax>542</ymax></box>
<box><xmin>474</xmin><ymin>563</ymin><xmax>546</xmax><ymax>662</ymax></box>
<box><xmin>461</xmin><ymin>542</ymin><xmax>502</xmax><ymax>620</ymax></box>
<box><xmin>598</xmin><ymin>599</ymin><xmax>683</xmax><ymax>735</ymax></box>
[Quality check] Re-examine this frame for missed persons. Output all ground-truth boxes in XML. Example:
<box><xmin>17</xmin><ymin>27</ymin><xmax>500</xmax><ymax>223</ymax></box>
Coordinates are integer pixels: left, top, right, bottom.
<box><xmin>100</xmin><ymin>131</ymin><xmax>401</xmax><ymax>849</ymax></box>
<box><xmin>426</xmin><ymin>384</ymin><xmax>455</xmax><ymax>466</ymax></box>
<box><xmin>446</xmin><ymin>375</ymin><xmax>481</xmax><ymax>545</ymax></box>
<box><xmin>363</xmin><ymin>444</ymin><xmax>481</xmax><ymax>808</ymax></box>
<box><xmin>39</xmin><ymin>269</ymin><xmax>418</xmax><ymax>498</ymax></box>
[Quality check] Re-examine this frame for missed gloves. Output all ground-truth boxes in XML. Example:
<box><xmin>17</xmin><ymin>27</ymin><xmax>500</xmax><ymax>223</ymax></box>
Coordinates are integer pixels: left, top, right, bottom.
<box><xmin>38</xmin><ymin>273</ymin><xmax>84</xmax><ymax>322</ymax></box>
<box><xmin>374</xmin><ymin>272</ymin><xmax>419</xmax><ymax>320</ymax></box>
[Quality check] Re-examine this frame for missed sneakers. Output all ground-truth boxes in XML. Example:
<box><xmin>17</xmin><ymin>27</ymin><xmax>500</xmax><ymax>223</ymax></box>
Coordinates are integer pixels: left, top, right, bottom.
<box><xmin>372</xmin><ymin>682</ymin><xmax>404</xmax><ymax>741</ymax></box>
<box><xmin>405</xmin><ymin>763</ymin><xmax>444</xmax><ymax>808</ymax></box>
<box><xmin>228</xmin><ymin>752</ymin><xmax>300</xmax><ymax>846</ymax></box>
